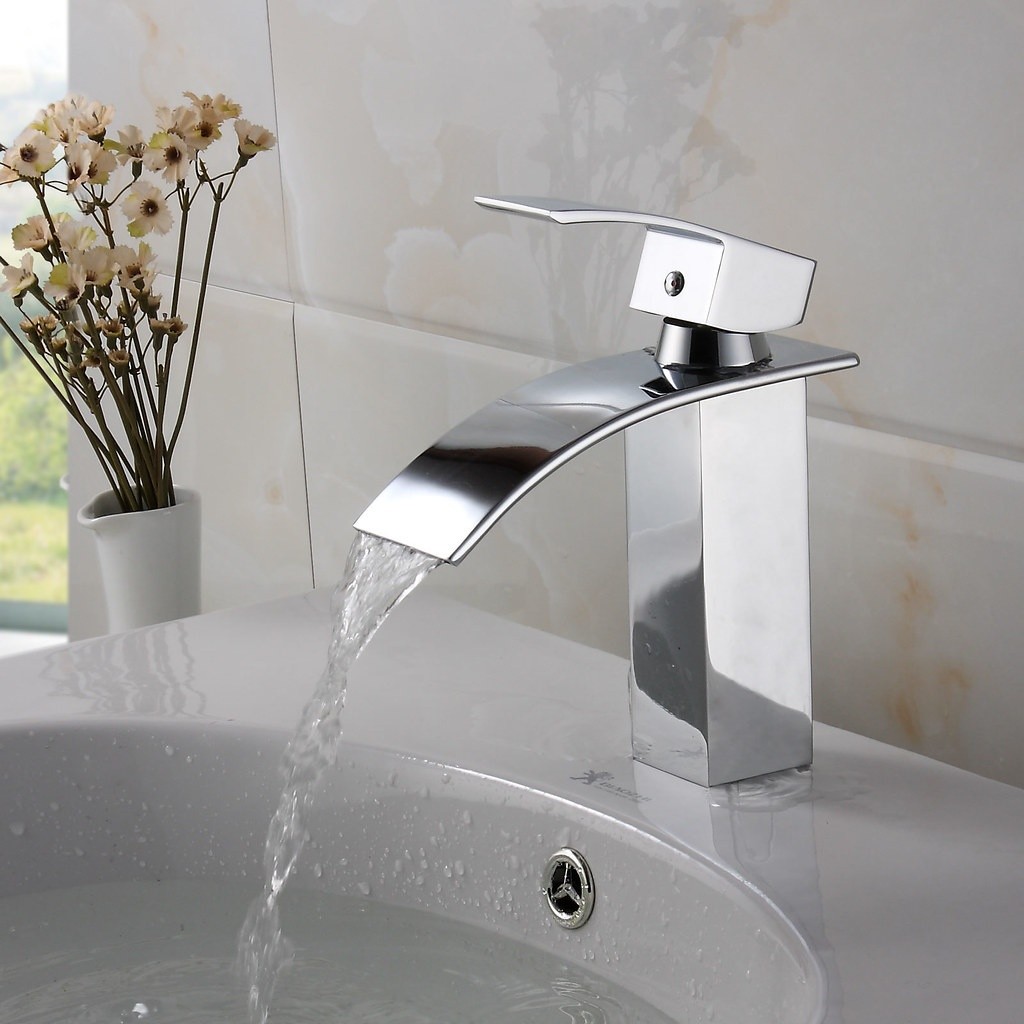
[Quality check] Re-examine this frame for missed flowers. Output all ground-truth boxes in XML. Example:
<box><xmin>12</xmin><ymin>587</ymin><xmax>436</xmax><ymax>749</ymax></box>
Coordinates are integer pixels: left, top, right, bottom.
<box><xmin>1</xmin><ymin>89</ymin><xmax>278</xmax><ymax>514</ymax></box>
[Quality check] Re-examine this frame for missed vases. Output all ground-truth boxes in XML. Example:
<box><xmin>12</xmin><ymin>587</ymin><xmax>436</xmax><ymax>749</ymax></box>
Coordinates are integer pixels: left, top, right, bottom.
<box><xmin>75</xmin><ymin>481</ymin><xmax>202</xmax><ymax>635</ymax></box>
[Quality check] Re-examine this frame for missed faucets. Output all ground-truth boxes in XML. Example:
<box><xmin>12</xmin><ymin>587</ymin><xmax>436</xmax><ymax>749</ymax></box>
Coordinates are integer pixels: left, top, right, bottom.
<box><xmin>353</xmin><ymin>195</ymin><xmax>861</xmax><ymax>787</ymax></box>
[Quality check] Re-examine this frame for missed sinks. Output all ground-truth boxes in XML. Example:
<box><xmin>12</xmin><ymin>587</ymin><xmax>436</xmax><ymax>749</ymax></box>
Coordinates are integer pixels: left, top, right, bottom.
<box><xmin>2</xmin><ymin>719</ymin><xmax>829</xmax><ymax>1023</ymax></box>
